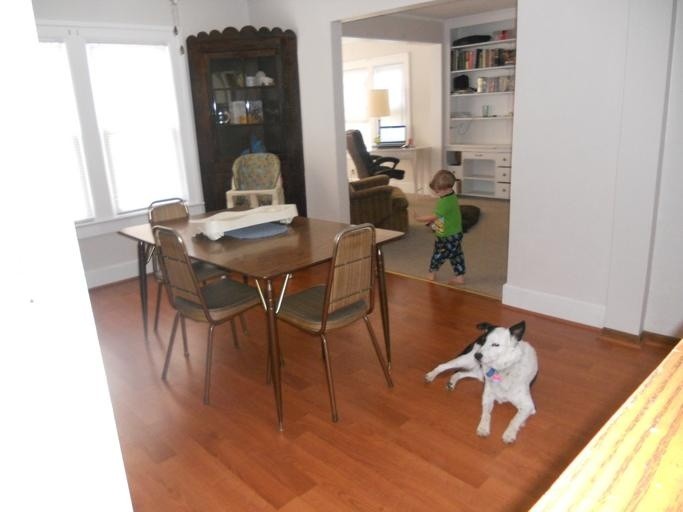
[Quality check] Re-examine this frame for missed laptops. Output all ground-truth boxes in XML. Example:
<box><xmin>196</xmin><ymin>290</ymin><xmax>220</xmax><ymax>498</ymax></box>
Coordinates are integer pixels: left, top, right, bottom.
<box><xmin>376</xmin><ymin>124</ymin><xmax>407</xmax><ymax>147</ymax></box>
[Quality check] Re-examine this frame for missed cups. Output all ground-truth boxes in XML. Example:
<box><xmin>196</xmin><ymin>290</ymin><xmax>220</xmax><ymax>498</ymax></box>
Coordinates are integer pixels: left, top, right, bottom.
<box><xmin>481</xmin><ymin>105</ymin><xmax>488</xmax><ymax>117</ymax></box>
<box><xmin>228</xmin><ymin>100</ymin><xmax>246</xmax><ymax>125</ymax></box>
<box><xmin>212</xmin><ymin>110</ymin><xmax>229</xmax><ymax>125</ymax></box>
<box><xmin>477</xmin><ymin>75</ymin><xmax>510</xmax><ymax>93</ymax></box>
<box><xmin>245</xmin><ymin>99</ymin><xmax>264</xmax><ymax>125</ymax></box>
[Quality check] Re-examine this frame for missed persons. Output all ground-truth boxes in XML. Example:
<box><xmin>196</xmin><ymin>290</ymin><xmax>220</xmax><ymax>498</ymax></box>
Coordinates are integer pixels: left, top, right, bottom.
<box><xmin>413</xmin><ymin>170</ymin><xmax>465</xmax><ymax>283</ymax></box>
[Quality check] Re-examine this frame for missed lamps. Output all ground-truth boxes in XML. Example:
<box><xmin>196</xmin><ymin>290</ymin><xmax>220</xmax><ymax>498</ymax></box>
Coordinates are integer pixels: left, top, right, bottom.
<box><xmin>367</xmin><ymin>90</ymin><xmax>390</xmax><ymax>144</ymax></box>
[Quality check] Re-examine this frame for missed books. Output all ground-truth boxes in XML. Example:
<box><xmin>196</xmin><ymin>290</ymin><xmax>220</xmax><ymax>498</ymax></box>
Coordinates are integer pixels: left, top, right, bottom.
<box><xmin>451</xmin><ymin>48</ymin><xmax>516</xmax><ymax>93</ymax></box>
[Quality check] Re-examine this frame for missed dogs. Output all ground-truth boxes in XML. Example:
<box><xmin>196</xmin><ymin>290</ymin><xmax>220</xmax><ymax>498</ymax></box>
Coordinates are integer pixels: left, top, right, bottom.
<box><xmin>425</xmin><ymin>321</ymin><xmax>539</xmax><ymax>444</ymax></box>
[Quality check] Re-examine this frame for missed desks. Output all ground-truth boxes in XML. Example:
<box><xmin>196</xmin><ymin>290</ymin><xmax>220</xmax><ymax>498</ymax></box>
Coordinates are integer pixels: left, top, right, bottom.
<box><xmin>369</xmin><ymin>146</ymin><xmax>431</xmax><ymax>199</ymax></box>
<box><xmin>116</xmin><ymin>206</ymin><xmax>406</xmax><ymax>432</ymax></box>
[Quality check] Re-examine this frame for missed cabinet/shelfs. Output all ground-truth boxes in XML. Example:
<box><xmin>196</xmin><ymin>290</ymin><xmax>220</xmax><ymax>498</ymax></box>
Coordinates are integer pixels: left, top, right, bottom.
<box><xmin>450</xmin><ymin>20</ymin><xmax>517</xmax><ymax>121</ymax></box>
<box><xmin>443</xmin><ymin>144</ymin><xmax>511</xmax><ymax>200</ymax></box>
<box><xmin>185</xmin><ymin>25</ymin><xmax>307</xmax><ymax>218</ymax></box>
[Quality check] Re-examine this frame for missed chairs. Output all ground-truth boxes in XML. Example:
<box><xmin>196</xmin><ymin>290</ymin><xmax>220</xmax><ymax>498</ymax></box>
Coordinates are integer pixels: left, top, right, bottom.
<box><xmin>344</xmin><ymin>129</ymin><xmax>404</xmax><ymax>180</ymax></box>
<box><xmin>142</xmin><ymin>198</ymin><xmax>253</xmax><ymax>335</ymax></box>
<box><xmin>347</xmin><ymin>174</ymin><xmax>408</xmax><ymax>231</ymax></box>
<box><xmin>265</xmin><ymin>221</ymin><xmax>395</xmax><ymax>423</ymax></box>
<box><xmin>151</xmin><ymin>223</ymin><xmax>284</xmax><ymax>406</ymax></box>
<box><xmin>222</xmin><ymin>152</ymin><xmax>289</xmax><ymax>214</ymax></box>
